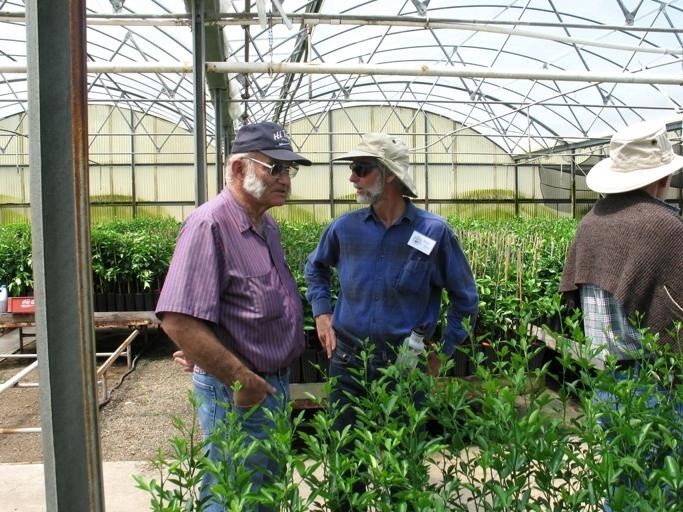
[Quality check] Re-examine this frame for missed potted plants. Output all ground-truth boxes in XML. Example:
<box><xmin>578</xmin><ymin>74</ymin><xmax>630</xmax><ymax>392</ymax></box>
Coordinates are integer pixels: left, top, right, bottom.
<box><xmin>0</xmin><ymin>213</ymin><xmax>175</xmax><ymax>313</ymax></box>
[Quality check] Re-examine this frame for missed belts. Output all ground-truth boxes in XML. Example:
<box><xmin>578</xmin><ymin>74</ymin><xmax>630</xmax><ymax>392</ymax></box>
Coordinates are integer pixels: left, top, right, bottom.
<box><xmin>604</xmin><ymin>359</ymin><xmax>652</xmax><ymax>372</ymax></box>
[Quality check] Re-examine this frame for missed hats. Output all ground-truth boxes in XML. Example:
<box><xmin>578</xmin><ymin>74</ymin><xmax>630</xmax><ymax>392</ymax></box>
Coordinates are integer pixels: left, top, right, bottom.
<box><xmin>585</xmin><ymin>121</ymin><xmax>682</xmax><ymax>194</ymax></box>
<box><xmin>329</xmin><ymin>131</ymin><xmax>417</xmax><ymax>195</ymax></box>
<box><xmin>229</xmin><ymin>123</ymin><xmax>312</xmax><ymax>165</ymax></box>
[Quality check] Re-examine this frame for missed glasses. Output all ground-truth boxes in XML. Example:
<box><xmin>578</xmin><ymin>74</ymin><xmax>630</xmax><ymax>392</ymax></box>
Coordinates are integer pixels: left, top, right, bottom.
<box><xmin>240</xmin><ymin>156</ymin><xmax>299</xmax><ymax>178</ymax></box>
<box><xmin>351</xmin><ymin>161</ymin><xmax>382</xmax><ymax>178</ymax></box>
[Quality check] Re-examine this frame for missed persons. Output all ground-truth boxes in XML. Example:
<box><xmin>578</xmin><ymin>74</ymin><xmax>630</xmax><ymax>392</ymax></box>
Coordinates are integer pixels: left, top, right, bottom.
<box><xmin>152</xmin><ymin>120</ymin><xmax>310</xmax><ymax>512</ymax></box>
<box><xmin>558</xmin><ymin>118</ymin><xmax>682</xmax><ymax>512</ymax></box>
<box><xmin>303</xmin><ymin>133</ymin><xmax>478</xmax><ymax>512</ymax></box>
